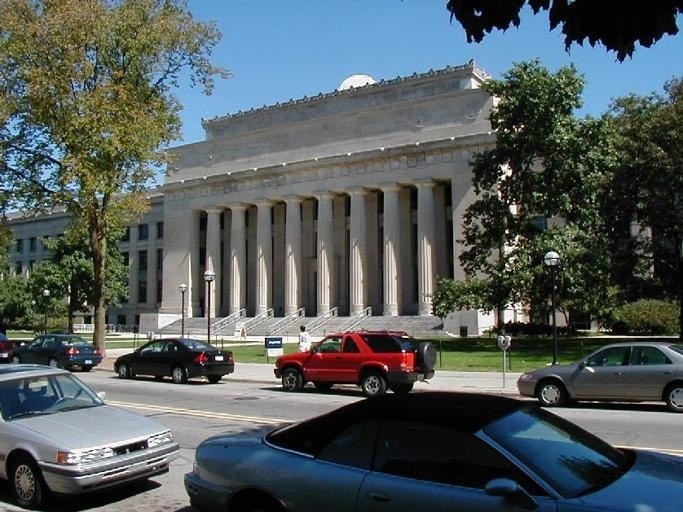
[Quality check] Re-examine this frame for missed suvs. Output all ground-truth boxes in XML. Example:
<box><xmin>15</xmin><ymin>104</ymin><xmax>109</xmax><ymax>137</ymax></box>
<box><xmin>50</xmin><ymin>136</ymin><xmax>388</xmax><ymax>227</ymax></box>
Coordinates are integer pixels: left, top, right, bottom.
<box><xmin>273</xmin><ymin>331</ymin><xmax>437</xmax><ymax>397</ymax></box>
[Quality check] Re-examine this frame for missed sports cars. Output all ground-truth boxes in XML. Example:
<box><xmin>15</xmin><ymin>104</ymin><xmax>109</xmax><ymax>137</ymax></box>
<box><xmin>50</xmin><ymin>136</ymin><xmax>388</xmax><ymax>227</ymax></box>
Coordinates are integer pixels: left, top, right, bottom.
<box><xmin>183</xmin><ymin>391</ymin><xmax>683</xmax><ymax>512</ymax></box>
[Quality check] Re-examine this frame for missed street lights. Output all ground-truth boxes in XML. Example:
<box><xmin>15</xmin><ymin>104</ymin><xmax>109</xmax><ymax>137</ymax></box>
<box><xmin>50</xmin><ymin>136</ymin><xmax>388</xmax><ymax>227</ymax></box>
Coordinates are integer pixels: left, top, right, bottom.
<box><xmin>542</xmin><ymin>251</ymin><xmax>560</xmax><ymax>367</ymax></box>
<box><xmin>42</xmin><ymin>288</ymin><xmax>49</xmax><ymax>334</ymax></box>
<box><xmin>180</xmin><ymin>282</ymin><xmax>187</xmax><ymax>341</ymax></box>
<box><xmin>203</xmin><ymin>270</ymin><xmax>214</xmax><ymax>350</ymax></box>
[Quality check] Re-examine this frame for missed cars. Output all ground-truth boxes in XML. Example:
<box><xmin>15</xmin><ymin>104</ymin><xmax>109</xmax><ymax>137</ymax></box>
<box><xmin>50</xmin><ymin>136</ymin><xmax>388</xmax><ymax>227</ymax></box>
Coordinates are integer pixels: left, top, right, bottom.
<box><xmin>8</xmin><ymin>334</ymin><xmax>102</xmax><ymax>371</ymax></box>
<box><xmin>114</xmin><ymin>339</ymin><xmax>234</xmax><ymax>383</ymax></box>
<box><xmin>0</xmin><ymin>364</ymin><xmax>180</xmax><ymax>507</ymax></box>
<box><xmin>516</xmin><ymin>343</ymin><xmax>681</xmax><ymax>412</ymax></box>
<box><xmin>0</xmin><ymin>332</ymin><xmax>14</xmax><ymax>363</ymax></box>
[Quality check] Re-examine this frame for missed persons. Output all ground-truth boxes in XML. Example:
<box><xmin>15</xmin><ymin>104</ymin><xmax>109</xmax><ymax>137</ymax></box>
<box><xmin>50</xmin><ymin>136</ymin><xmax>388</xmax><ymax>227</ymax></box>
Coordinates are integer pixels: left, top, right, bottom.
<box><xmin>297</xmin><ymin>326</ymin><xmax>311</xmax><ymax>352</ymax></box>
